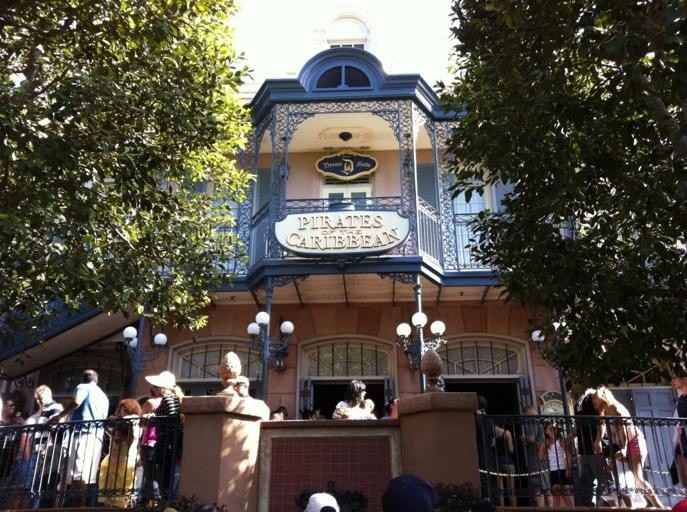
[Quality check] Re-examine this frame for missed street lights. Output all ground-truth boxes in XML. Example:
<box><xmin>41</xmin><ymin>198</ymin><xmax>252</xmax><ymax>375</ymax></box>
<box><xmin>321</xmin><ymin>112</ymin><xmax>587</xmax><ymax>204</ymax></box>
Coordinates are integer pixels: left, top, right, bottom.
<box><xmin>529</xmin><ymin>322</ymin><xmax>609</xmax><ymax>492</ymax></box>
<box><xmin>247</xmin><ymin>286</ymin><xmax>295</xmax><ymax>407</ymax></box>
<box><xmin>122</xmin><ymin>301</ymin><xmax>168</xmax><ymax>401</ymax></box>
<box><xmin>398</xmin><ymin>284</ymin><xmax>446</xmax><ymax>393</ymax></box>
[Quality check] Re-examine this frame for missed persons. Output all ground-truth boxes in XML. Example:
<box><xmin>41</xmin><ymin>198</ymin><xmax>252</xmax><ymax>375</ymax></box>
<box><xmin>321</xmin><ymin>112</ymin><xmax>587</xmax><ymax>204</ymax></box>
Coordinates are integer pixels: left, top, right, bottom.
<box><xmin>0</xmin><ymin>366</ymin><xmax>185</xmax><ymax>512</ymax></box>
<box><xmin>270</xmin><ymin>379</ymin><xmax>402</xmax><ymax>426</ymax></box>
<box><xmin>472</xmin><ymin>362</ymin><xmax>687</xmax><ymax>512</ymax></box>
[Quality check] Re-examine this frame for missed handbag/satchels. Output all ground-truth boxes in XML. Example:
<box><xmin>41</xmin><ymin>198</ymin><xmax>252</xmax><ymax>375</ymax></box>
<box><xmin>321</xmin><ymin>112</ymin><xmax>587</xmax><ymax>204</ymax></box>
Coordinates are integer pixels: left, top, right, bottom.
<box><xmin>600</xmin><ymin>436</ymin><xmax>623</xmax><ymax>459</ymax></box>
<box><xmin>625</xmin><ymin>437</ymin><xmax>640</xmax><ymax>458</ymax></box>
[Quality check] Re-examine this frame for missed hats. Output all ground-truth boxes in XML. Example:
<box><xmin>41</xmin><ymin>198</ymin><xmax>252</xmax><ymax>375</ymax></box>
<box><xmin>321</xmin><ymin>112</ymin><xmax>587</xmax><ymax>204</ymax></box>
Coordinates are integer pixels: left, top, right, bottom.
<box><xmin>80</xmin><ymin>369</ymin><xmax>98</xmax><ymax>380</ymax></box>
<box><xmin>382</xmin><ymin>472</ymin><xmax>438</xmax><ymax>512</ymax></box>
<box><xmin>144</xmin><ymin>370</ymin><xmax>177</xmax><ymax>391</ymax></box>
<box><xmin>304</xmin><ymin>493</ymin><xmax>341</xmax><ymax>512</ymax></box>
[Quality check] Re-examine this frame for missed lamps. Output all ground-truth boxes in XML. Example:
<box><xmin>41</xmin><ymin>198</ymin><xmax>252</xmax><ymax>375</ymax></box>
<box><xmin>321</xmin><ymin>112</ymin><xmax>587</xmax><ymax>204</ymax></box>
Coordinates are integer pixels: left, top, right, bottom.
<box><xmin>123</xmin><ymin>326</ymin><xmax>168</xmax><ymax>375</ymax></box>
<box><xmin>532</xmin><ymin>320</ymin><xmax>579</xmax><ymax>367</ymax></box>
<box><xmin>395</xmin><ymin>312</ymin><xmax>448</xmax><ymax>376</ymax></box>
<box><xmin>408</xmin><ymin>339</ymin><xmax>420</xmax><ymax>372</ymax></box>
<box><xmin>276</xmin><ymin>350</ymin><xmax>285</xmax><ymax>371</ymax></box>
<box><xmin>247</xmin><ymin>311</ymin><xmax>295</xmax><ymax>372</ymax></box>
